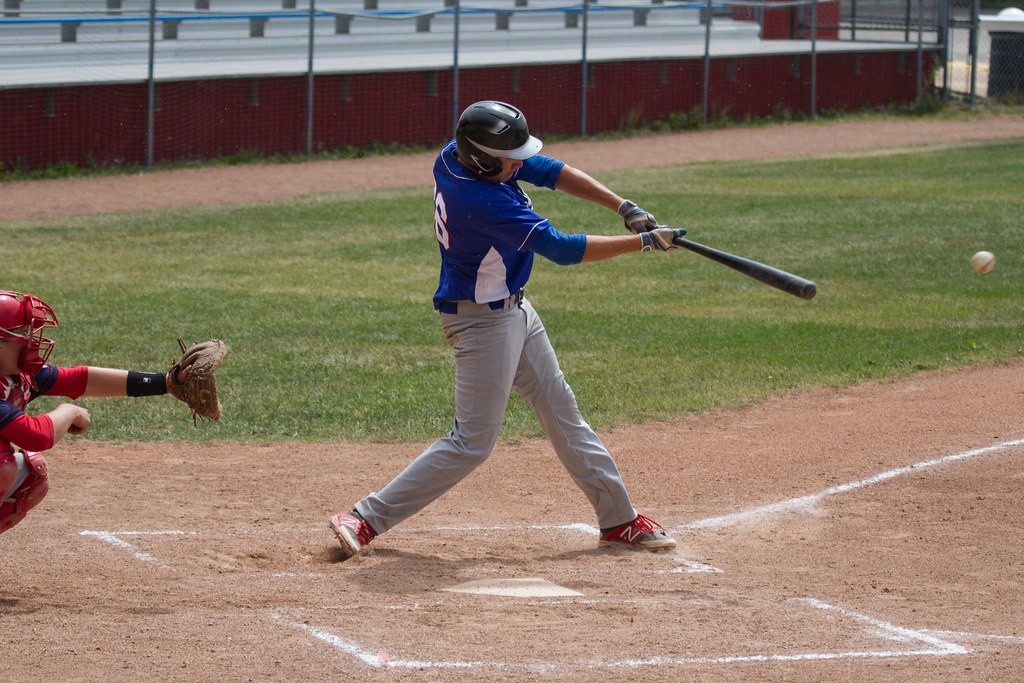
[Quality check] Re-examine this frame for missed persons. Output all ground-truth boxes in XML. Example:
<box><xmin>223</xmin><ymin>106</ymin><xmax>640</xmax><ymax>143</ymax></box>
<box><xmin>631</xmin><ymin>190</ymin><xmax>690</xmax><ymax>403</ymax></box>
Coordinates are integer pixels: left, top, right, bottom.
<box><xmin>0</xmin><ymin>288</ymin><xmax>192</xmax><ymax>531</ymax></box>
<box><xmin>328</xmin><ymin>100</ymin><xmax>687</xmax><ymax>556</ymax></box>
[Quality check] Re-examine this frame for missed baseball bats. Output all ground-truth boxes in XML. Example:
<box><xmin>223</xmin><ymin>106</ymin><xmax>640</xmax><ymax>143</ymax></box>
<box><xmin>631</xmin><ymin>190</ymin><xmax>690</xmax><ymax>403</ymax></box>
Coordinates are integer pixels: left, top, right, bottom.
<box><xmin>624</xmin><ymin>222</ymin><xmax>817</xmax><ymax>300</ymax></box>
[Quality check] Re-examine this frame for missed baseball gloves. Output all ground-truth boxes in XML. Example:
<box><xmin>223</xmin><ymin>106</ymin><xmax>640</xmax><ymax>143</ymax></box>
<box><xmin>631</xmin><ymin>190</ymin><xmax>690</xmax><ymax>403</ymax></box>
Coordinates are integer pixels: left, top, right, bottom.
<box><xmin>168</xmin><ymin>338</ymin><xmax>227</xmax><ymax>420</ymax></box>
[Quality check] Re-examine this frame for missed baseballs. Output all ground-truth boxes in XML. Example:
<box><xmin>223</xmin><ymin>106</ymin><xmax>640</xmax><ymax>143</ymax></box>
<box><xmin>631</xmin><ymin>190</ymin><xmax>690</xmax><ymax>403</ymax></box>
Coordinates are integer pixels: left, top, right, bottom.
<box><xmin>971</xmin><ymin>251</ymin><xmax>996</xmax><ymax>275</ymax></box>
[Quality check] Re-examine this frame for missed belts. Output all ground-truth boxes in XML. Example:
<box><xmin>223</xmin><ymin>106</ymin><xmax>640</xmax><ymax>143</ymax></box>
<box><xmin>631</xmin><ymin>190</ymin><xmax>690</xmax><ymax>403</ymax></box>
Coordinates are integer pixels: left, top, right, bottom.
<box><xmin>442</xmin><ymin>287</ymin><xmax>524</xmax><ymax>315</ymax></box>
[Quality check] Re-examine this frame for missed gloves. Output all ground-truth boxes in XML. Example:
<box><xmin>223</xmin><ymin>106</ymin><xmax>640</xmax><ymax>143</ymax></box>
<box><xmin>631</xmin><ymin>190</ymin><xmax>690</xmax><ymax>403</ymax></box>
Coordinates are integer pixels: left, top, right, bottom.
<box><xmin>618</xmin><ymin>199</ymin><xmax>658</xmax><ymax>233</ymax></box>
<box><xmin>639</xmin><ymin>225</ymin><xmax>687</xmax><ymax>252</ymax></box>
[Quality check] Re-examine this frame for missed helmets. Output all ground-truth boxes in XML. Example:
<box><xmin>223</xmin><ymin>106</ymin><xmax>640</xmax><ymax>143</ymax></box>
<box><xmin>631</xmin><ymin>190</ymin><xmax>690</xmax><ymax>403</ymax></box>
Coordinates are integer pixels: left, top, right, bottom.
<box><xmin>0</xmin><ymin>289</ymin><xmax>26</xmax><ymax>332</ymax></box>
<box><xmin>456</xmin><ymin>100</ymin><xmax>544</xmax><ymax>175</ymax></box>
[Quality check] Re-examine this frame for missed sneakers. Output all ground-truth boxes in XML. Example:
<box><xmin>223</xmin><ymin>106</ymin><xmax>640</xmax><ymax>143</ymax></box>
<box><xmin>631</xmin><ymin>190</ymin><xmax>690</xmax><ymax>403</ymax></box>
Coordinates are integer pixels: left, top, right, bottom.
<box><xmin>329</xmin><ymin>509</ymin><xmax>377</xmax><ymax>557</ymax></box>
<box><xmin>598</xmin><ymin>514</ymin><xmax>677</xmax><ymax>551</ymax></box>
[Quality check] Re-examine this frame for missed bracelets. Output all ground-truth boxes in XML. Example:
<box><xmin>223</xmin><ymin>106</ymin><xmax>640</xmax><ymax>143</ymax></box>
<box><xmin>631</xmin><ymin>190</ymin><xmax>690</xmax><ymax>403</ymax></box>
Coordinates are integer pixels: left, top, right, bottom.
<box><xmin>125</xmin><ymin>369</ymin><xmax>168</xmax><ymax>396</ymax></box>
